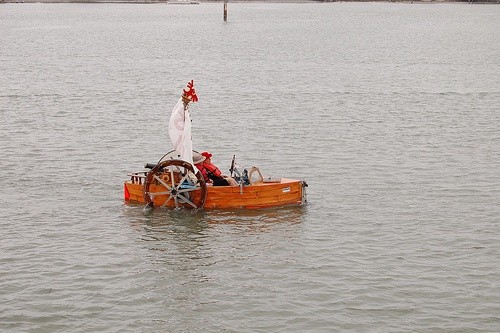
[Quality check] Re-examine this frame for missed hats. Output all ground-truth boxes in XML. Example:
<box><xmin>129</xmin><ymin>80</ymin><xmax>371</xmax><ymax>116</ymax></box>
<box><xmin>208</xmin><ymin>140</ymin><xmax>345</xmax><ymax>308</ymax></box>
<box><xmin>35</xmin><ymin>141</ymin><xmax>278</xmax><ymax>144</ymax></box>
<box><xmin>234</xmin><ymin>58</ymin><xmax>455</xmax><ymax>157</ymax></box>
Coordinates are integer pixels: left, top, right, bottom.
<box><xmin>202</xmin><ymin>152</ymin><xmax>212</xmax><ymax>160</ymax></box>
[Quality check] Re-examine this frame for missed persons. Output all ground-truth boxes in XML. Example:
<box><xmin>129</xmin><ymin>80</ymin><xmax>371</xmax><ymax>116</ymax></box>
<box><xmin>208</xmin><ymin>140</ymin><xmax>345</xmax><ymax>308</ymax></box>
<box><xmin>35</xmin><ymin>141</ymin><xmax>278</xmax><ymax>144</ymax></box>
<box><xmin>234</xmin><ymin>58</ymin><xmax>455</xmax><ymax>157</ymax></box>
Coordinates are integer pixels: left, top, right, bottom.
<box><xmin>192</xmin><ymin>151</ymin><xmax>239</xmax><ymax>186</ymax></box>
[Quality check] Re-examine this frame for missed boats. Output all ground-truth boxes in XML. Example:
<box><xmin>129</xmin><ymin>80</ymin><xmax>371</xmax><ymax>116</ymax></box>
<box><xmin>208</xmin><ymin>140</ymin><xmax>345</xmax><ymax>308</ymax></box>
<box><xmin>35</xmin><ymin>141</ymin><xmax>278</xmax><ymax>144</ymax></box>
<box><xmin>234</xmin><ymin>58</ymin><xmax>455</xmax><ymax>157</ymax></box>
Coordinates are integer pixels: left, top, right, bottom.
<box><xmin>123</xmin><ymin>79</ymin><xmax>309</xmax><ymax>217</ymax></box>
